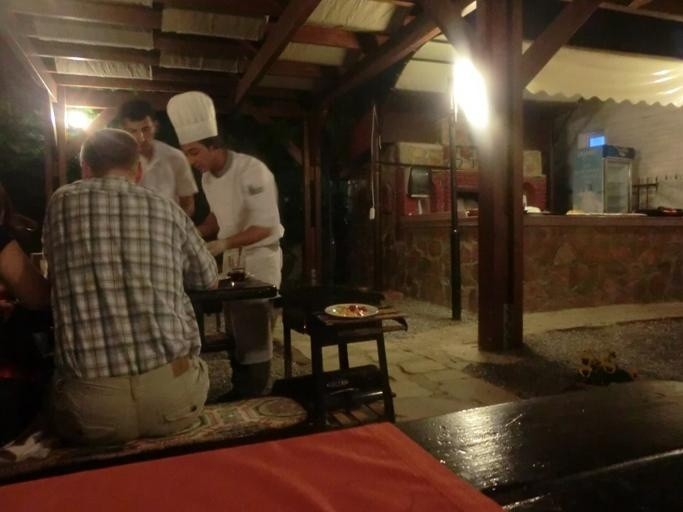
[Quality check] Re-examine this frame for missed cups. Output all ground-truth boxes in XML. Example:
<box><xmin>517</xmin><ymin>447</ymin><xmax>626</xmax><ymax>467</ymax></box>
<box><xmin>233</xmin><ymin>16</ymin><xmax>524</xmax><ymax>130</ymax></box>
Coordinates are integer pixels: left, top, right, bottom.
<box><xmin>226</xmin><ymin>254</ymin><xmax>247</xmax><ymax>281</ymax></box>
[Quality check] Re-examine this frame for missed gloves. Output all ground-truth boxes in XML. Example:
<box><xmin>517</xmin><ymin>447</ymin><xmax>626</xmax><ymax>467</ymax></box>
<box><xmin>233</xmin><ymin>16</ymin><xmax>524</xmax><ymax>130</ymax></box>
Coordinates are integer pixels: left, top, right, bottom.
<box><xmin>206</xmin><ymin>240</ymin><xmax>225</xmax><ymax>256</ymax></box>
<box><xmin>0</xmin><ymin>429</ymin><xmax>54</xmax><ymax>464</ymax></box>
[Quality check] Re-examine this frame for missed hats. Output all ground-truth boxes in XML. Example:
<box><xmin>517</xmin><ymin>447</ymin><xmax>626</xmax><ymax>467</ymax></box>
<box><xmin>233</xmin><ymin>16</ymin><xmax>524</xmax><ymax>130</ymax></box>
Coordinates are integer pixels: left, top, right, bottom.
<box><xmin>165</xmin><ymin>90</ymin><xmax>218</xmax><ymax>145</ymax></box>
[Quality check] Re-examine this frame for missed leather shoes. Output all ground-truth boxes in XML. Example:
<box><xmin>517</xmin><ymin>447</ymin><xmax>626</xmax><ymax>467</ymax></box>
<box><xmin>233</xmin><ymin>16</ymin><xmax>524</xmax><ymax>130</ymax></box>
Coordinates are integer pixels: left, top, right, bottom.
<box><xmin>217</xmin><ymin>389</ymin><xmax>254</xmax><ymax>402</ymax></box>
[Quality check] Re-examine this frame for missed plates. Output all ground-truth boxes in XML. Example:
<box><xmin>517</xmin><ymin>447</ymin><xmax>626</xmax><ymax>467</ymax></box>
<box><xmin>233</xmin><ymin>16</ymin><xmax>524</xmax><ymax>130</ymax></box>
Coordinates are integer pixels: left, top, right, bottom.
<box><xmin>323</xmin><ymin>302</ymin><xmax>380</xmax><ymax>319</ymax></box>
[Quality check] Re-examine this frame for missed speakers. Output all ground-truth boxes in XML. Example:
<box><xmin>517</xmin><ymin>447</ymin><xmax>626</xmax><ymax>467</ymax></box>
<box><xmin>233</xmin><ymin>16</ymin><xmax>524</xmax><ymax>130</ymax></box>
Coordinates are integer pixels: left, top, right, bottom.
<box><xmin>408</xmin><ymin>166</ymin><xmax>432</xmax><ymax>199</ymax></box>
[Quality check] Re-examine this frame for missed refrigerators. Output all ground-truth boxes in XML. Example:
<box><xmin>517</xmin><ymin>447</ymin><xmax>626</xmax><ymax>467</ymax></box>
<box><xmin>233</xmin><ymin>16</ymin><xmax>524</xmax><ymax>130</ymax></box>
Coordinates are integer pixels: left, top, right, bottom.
<box><xmin>570</xmin><ymin>143</ymin><xmax>638</xmax><ymax>216</ymax></box>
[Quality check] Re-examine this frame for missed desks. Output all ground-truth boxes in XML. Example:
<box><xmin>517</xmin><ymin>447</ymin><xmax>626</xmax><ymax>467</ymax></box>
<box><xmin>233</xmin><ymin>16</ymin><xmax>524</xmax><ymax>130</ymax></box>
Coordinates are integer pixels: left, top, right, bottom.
<box><xmin>0</xmin><ymin>270</ymin><xmax>277</xmax><ymax>351</ymax></box>
<box><xmin>277</xmin><ymin>284</ymin><xmax>409</xmax><ymax>431</ymax></box>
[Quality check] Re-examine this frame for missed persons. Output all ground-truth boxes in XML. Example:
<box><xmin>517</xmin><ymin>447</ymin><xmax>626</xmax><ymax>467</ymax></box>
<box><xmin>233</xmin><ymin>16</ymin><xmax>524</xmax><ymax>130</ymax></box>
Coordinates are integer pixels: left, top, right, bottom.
<box><xmin>0</xmin><ymin>209</ymin><xmax>60</xmax><ymax>465</ymax></box>
<box><xmin>165</xmin><ymin>89</ymin><xmax>290</xmax><ymax>406</ymax></box>
<box><xmin>36</xmin><ymin>125</ymin><xmax>221</xmax><ymax>450</ymax></box>
<box><xmin>109</xmin><ymin>98</ymin><xmax>198</xmax><ymax>225</ymax></box>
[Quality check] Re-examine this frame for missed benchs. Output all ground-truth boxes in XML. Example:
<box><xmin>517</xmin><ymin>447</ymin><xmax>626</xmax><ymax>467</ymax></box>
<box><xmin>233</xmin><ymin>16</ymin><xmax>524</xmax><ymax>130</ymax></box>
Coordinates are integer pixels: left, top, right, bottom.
<box><xmin>1</xmin><ymin>376</ymin><xmax>682</xmax><ymax>511</ymax></box>
<box><xmin>1</xmin><ymin>395</ymin><xmax>309</xmax><ymax>479</ymax></box>
